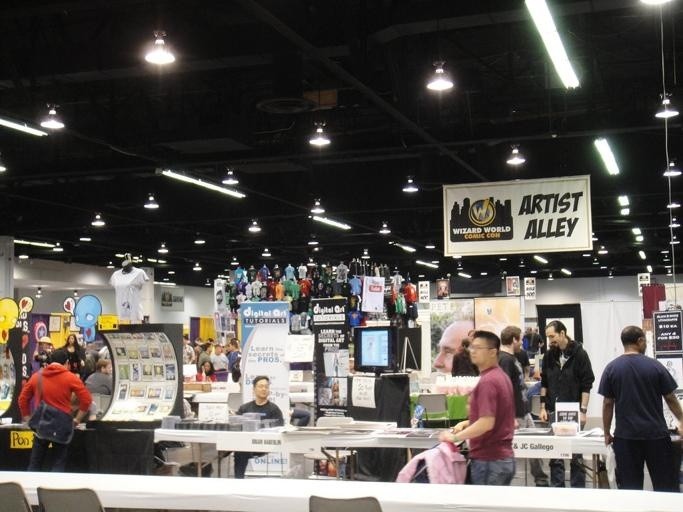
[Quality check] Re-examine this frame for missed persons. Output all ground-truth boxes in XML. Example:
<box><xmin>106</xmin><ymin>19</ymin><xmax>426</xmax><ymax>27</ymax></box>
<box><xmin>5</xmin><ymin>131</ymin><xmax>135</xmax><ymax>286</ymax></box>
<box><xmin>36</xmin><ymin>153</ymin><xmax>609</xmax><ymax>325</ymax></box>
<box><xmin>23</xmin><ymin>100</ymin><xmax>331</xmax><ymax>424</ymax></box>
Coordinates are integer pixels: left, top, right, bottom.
<box><xmin>153</xmin><ymin>398</ymin><xmax>193</xmax><ymax>469</ymax></box>
<box><xmin>209</xmin><ymin>344</ymin><xmax>229</xmax><ymax>371</ymax></box>
<box><xmin>227</xmin><ymin>342</ymin><xmax>239</xmax><ymax>373</ymax></box>
<box><xmin>198</xmin><ymin>342</ymin><xmax>213</xmax><ymax>370</ymax></box>
<box><xmin>183</xmin><ymin>335</ymin><xmax>196</xmax><ymax>382</ymax></box>
<box><xmin>434</xmin><ymin>320</ymin><xmax>474</xmax><ymax>374</ymax></box>
<box><xmin>197</xmin><ymin>359</ymin><xmax>217</xmax><ymax>382</ymax></box>
<box><xmin>17</xmin><ymin>349</ymin><xmax>92</xmax><ymax>473</ymax></box>
<box><xmin>194</xmin><ymin>337</ymin><xmax>206</xmax><ymax>364</ymax></box>
<box><xmin>33</xmin><ymin>337</ymin><xmax>55</xmax><ymax>370</ymax></box>
<box><xmin>85</xmin><ymin>341</ymin><xmax>98</xmax><ymax>364</ymax></box>
<box><xmin>452</xmin><ymin>339</ymin><xmax>480</xmax><ymax>376</ymax></box>
<box><xmin>598</xmin><ymin>326</ymin><xmax>683</xmax><ymax>493</ymax></box>
<box><xmin>59</xmin><ymin>334</ymin><xmax>84</xmax><ymax>378</ymax></box>
<box><xmin>525</xmin><ymin>372</ymin><xmax>543</xmax><ymax>420</ymax></box>
<box><xmin>289</xmin><ymin>396</ymin><xmax>310</xmax><ymax>426</ymax></box>
<box><xmin>498</xmin><ymin>326</ymin><xmax>551</xmax><ymax>487</ymax></box>
<box><xmin>84</xmin><ymin>359</ymin><xmax>113</xmax><ymax>395</ymax></box>
<box><xmin>232</xmin><ymin>356</ymin><xmax>242</xmax><ymax>383</ymax></box>
<box><xmin>220</xmin><ymin>376</ymin><xmax>284</xmax><ymax>480</ymax></box>
<box><xmin>514</xmin><ymin>349</ymin><xmax>531</xmax><ymax>383</ymax></box>
<box><xmin>529</xmin><ymin>329</ymin><xmax>544</xmax><ymax>359</ymax></box>
<box><xmin>526</xmin><ymin>327</ymin><xmax>533</xmax><ymax>359</ymax></box>
<box><xmin>438</xmin><ymin>331</ymin><xmax>516</xmax><ymax>486</ymax></box>
<box><xmin>225</xmin><ymin>338</ymin><xmax>242</xmax><ymax>357</ymax></box>
<box><xmin>540</xmin><ymin>321</ymin><xmax>595</xmax><ymax>488</ymax></box>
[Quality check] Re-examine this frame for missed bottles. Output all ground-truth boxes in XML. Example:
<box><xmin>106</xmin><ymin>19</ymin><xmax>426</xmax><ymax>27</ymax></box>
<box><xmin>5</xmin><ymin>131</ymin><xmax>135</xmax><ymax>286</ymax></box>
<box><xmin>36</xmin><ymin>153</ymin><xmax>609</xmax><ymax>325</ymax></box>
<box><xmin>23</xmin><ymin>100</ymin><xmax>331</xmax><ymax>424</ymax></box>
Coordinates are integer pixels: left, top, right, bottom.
<box><xmin>415</xmin><ymin>403</ymin><xmax>424</xmax><ymax>428</ymax></box>
<box><xmin>202</xmin><ymin>369</ymin><xmax>206</xmax><ymax>382</ymax></box>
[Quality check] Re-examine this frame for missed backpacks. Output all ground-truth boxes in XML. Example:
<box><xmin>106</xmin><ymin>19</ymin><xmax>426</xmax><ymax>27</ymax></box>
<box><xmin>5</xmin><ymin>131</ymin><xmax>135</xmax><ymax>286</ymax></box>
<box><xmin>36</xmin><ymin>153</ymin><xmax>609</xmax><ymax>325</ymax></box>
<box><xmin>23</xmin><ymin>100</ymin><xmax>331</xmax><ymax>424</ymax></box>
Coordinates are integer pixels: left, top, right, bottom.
<box><xmin>28</xmin><ymin>400</ymin><xmax>74</xmax><ymax>452</ymax></box>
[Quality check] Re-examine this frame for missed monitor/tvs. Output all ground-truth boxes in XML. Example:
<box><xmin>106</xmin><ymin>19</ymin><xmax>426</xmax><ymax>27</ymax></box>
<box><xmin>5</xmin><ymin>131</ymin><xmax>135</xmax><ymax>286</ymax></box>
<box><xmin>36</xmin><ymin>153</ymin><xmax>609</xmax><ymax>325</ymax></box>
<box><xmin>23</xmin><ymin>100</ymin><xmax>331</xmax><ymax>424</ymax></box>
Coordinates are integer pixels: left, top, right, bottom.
<box><xmin>353</xmin><ymin>326</ymin><xmax>400</xmax><ymax>376</ymax></box>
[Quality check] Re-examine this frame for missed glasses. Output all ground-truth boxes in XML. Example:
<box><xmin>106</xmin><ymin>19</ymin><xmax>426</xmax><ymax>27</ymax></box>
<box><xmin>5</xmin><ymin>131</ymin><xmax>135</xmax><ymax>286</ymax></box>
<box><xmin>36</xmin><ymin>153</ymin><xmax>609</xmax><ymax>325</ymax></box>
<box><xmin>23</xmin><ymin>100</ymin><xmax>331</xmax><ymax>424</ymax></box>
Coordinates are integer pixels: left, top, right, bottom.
<box><xmin>469</xmin><ymin>343</ymin><xmax>492</xmax><ymax>350</ymax></box>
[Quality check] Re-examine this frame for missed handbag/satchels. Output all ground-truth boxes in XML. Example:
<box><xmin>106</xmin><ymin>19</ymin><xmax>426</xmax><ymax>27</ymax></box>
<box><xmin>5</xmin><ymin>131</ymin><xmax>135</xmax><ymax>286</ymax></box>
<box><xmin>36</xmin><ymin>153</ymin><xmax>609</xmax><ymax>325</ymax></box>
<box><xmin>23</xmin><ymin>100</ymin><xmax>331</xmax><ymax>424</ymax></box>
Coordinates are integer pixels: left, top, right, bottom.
<box><xmin>28</xmin><ymin>367</ymin><xmax>74</xmax><ymax>445</ymax></box>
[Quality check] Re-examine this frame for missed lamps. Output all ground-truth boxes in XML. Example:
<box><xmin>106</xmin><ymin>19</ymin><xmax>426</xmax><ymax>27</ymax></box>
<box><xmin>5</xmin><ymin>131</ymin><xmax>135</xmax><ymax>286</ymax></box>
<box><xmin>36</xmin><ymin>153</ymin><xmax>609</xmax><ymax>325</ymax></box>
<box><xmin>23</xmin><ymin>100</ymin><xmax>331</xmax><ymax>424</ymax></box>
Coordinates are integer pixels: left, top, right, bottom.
<box><xmin>155</xmin><ymin>166</ymin><xmax>249</xmax><ymax>199</ymax></box>
<box><xmin>307</xmin><ymin>235</ymin><xmax>319</xmax><ymax>245</ymax></box>
<box><xmin>0</xmin><ymin>114</ymin><xmax>50</xmax><ymax>137</ymax></box>
<box><xmin>594</xmin><ymin>138</ymin><xmax>619</xmax><ymax>175</ymax></box>
<box><xmin>14</xmin><ymin>239</ymin><xmax>56</xmax><ymax>248</ymax></box>
<box><xmin>248</xmin><ymin>218</ymin><xmax>262</xmax><ymax>232</ymax></box>
<box><xmin>402</xmin><ymin>176</ymin><xmax>419</xmax><ymax>192</ymax></box>
<box><xmin>91</xmin><ymin>211</ymin><xmax>105</xmax><ymax>226</ymax></box>
<box><xmin>310</xmin><ymin>194</ymin><xmax>325</xmax><ymax>214</ymax></box>
<box><xmin>524</xmin><ymin>0</ymin><xmax>581</xmax><ymax>90</ymax></box>
<box><xmin>654</xmin><ymin>92</ymin><xmax>680</xmax><ymax>118</ymax></box>
<box><xmin>157</xmin><ymin>240</ymin><xmax>169</xmax><ymax>253</ymax></box>
<box><xmin>308</xmin><ymin>120</ymin><xmax>331</xmax><ymax>146</ymax></box>
<box><xmin>307</xmin><ymin>215</ymin><xmax>352</xmax><ymax>231</ymax></box>
<box><xmin>262</xmin><ymin>248</ymin><xmax>271</xmax><ymax>256</ymax></box>
<box><xmin>193</xmin><ymin>262</ymin><xmax>202</xmax><ymax>271</ymax></box>
<box><xmin>426</xmin><ymin>60</ymin><xmax>455</xmax><ymax>91</ymax></box>
<box><xmin>53</xmin><ymin>240</ymin><xmax>64</xmax><ymax>252</ymax></box>
<box><xmin>145</xmin><ymin>22</ymin><xmax>176</xmax><ymax>66</ymax></box>
<box><xmin>231</xmin><ymin>256</ymin><xmax>239</xmax><ymax>265</ymax></box>
<box><xmin>221</xmin><ymin>164</ymin><xmax>239</xmax><ymax>185</ymax></box>
<box><xmin>379</xmin><ymin>220</ymin><xmax>392</xmax><ymax>234</ymax></box>
<box><xmin>40</xmin><ymin>102</ymin><xmax>65</xmax><ymax>129</ymax></box>
<box><xmin>143</xmin><ymin>193</ymin><xmax>159</xmax><ymax>209</ymax></box>
<box><xmin>506</xmin><ymin>143</ymin><xmax>526</xmax><ymax>164</ymax></box>
